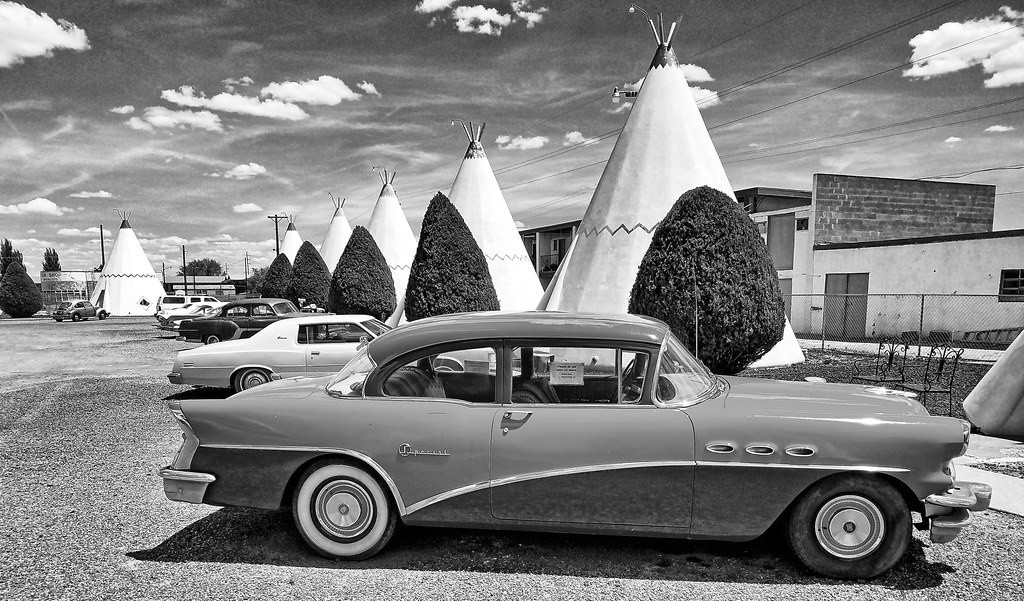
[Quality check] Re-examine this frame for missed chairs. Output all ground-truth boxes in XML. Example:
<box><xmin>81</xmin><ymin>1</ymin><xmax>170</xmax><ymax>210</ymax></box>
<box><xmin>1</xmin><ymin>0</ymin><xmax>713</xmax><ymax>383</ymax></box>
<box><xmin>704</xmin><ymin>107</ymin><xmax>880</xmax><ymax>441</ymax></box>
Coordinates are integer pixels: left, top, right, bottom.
<box><xmin>512</xmin><ymin>377</ymin><xmax>561</xmax><ymax>403</ymax></box>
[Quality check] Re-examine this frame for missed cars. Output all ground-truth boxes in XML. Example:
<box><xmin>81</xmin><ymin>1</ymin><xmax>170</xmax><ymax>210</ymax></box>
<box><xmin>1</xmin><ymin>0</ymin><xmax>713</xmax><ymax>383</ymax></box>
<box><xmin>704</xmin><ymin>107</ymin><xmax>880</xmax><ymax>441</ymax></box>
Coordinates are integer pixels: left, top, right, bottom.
<box><xmin>163</xmin><ymin>313</ymin><xmax>556</xmax><ymax>392</ymax></box>
<box><xmin>50</xmin><ymin>298</ymin><xmax>110</xmax><ymax>322</ymax></box>
<box><xmin>153</xmin><ymin>293</ymin><xmax>338</xmax><ymax>345</ymax></box>
<box><xmin>157</xmin><ymin>309</ymin><xmax>995</xmax><ymax>580</ymax></box>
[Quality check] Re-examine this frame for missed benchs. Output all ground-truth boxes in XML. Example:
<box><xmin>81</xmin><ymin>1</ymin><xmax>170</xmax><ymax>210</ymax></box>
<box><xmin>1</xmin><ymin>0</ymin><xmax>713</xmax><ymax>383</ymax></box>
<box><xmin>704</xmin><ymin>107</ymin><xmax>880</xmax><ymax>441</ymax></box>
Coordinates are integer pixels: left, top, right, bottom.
<box><xmin>384</xmin><ymin>366</ymin><xmax>446</xmax><ymax>398</ymax></box>
